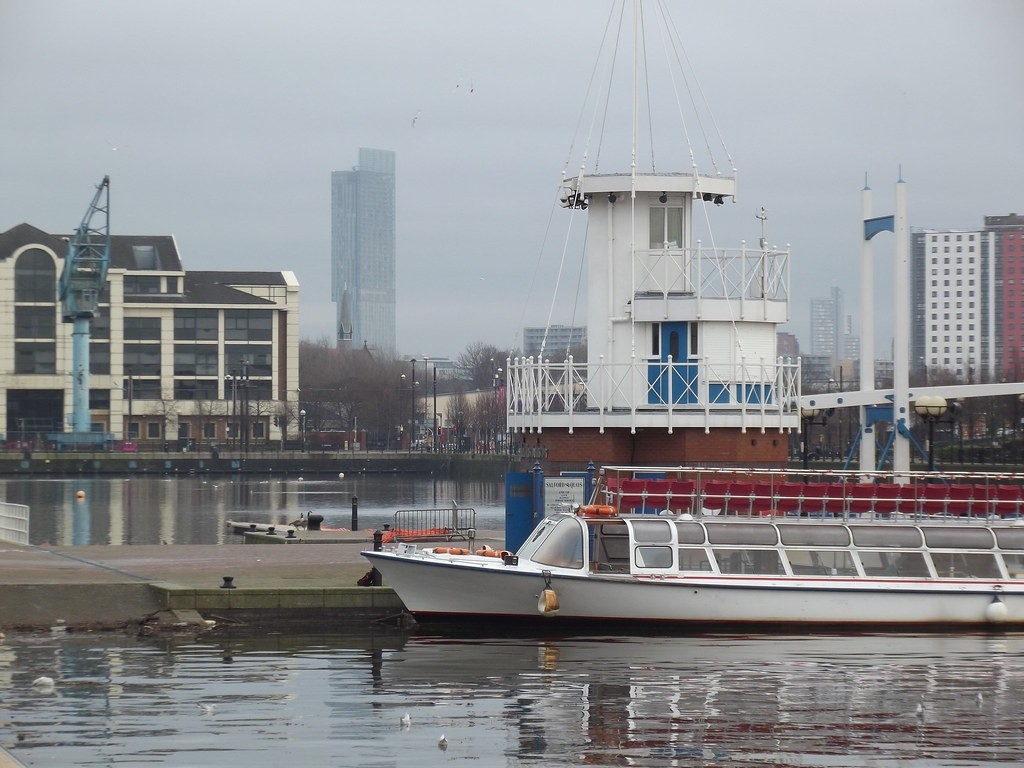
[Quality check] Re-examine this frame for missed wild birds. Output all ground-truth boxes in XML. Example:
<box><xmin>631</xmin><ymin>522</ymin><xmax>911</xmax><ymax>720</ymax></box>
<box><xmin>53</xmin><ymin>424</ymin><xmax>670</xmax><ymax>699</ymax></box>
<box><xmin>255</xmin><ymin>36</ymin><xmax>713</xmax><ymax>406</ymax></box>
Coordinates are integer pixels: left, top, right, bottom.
<box><xmin>470</xmin><ymin>88</ymin><xmax>474</xmax><ymax>92</ymax></box>
<box><xmin>32</xmin><ymin>676</ymin><xmax>55</xmax><ymax>686</ymax></box>
<box><xmin>914</xmin><ymin>692</ymin><xmax>928</xmax><ymax>720</ymax></box>
<box><xmin>439</xmin><ymin>734</ymin><xmax>448</xmax><ymax>746</ymax></box>
<box><xmin>974</xmin><ymin>692</ymin><xmax>984</xmax><ymax>702</ymax></box>
<box><xmin>456</xmin><ymin>83</ymin><xmax>460</xmax><ymax>90</ymax></box>
<box><xmin>410</xmin><ymin>110</ymin><xmax>422</xmax><ymax>127</ymax></box>
<box><xmin>399</xmin><ymin>713</ymin><xmax>411</xmax><ymax>726</ymax></box>
<box><xmin>196</xmin><ymin>700</ymin><xmax>219</xmax><ymax>713</ymax></box>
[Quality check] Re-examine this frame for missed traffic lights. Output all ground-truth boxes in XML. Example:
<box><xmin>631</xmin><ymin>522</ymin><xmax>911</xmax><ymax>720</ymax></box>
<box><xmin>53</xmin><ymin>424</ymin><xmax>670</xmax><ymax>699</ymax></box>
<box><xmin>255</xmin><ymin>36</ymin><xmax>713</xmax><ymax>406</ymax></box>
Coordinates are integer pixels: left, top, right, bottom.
<box><xmin>437</xmin><ymin>425</ymin><xmax>442</xmax><ymax>436</ymax></box>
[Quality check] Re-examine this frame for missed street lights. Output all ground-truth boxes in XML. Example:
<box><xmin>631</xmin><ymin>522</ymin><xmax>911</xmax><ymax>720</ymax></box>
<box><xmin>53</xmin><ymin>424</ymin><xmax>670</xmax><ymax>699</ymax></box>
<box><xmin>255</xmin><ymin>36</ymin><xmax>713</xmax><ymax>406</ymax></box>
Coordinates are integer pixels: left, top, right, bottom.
<box><xmin>299</xmin><ymin>408</ymin><xmax>307</xmax><ymax>452</ymax></box>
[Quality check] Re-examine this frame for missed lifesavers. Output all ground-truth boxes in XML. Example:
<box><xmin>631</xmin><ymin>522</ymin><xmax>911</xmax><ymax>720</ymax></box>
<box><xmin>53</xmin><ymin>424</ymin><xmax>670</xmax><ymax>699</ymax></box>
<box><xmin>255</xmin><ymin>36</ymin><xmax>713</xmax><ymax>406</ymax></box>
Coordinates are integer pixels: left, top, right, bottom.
<box><xmin>581</xmin><ymin>504</ymin><xmax>616</xmax><ymax>519</ymax></box>
<box><xmin>475</xmin><ymin>549</ymin><xmax>500</xmax><ymax>557</ymax></box>
<box><xmin>432</xmin><ymin>547</ymin><xmax>470</xmax><ymax>555</ymax></box>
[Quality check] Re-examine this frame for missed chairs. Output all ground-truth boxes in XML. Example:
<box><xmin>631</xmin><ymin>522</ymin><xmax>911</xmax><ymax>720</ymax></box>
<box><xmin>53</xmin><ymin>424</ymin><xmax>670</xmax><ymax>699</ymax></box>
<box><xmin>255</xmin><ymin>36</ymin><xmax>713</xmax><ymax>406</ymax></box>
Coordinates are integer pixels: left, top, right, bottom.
<box><xmin>605</xmin><ymin>476</ymin><xmax>1024</xmax><ymax>517</ymax></box>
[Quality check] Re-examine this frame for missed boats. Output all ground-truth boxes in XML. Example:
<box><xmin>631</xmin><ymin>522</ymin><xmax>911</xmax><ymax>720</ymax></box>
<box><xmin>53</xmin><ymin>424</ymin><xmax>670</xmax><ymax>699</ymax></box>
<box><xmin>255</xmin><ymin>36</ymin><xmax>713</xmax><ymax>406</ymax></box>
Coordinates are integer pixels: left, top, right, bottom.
<box><xmin>359</xmin><ymin>505</ymin><xmax>1024</xmax><ymax>630</ymax></box>
